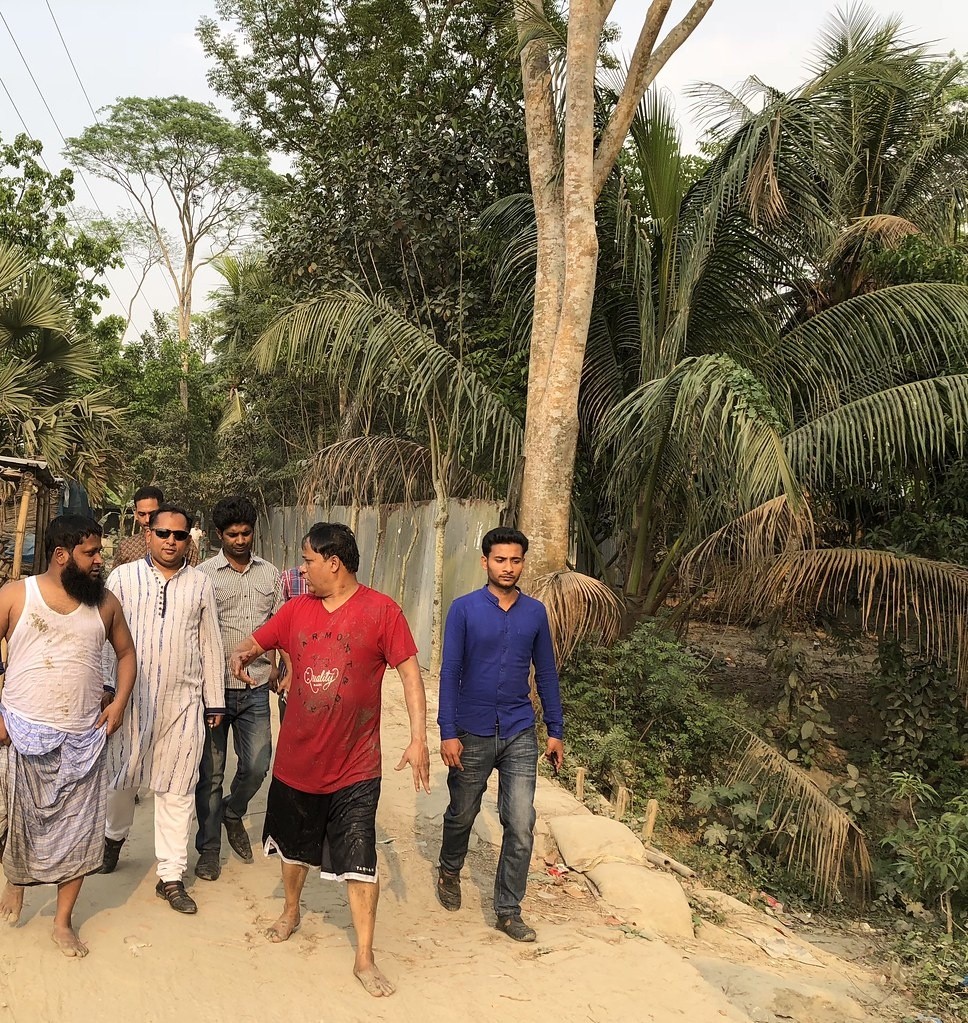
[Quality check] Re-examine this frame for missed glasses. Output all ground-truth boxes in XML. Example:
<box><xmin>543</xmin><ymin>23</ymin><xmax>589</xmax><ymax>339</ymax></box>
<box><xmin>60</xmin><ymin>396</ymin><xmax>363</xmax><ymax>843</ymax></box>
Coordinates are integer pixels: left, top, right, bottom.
<box><xmin>149</xmin><ymin>528</ymin><xmax>189</xmax><ymax>541</ymax></box>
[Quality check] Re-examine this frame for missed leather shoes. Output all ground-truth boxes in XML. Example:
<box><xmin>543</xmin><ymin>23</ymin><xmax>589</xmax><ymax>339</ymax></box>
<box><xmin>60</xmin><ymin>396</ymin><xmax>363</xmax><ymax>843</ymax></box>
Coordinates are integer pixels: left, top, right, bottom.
<box><xmin>195</xmin><ymin>852</ymin><xmax>221</xmax><ymax>880</ymax></box>
<box><xmin>221</xmin><ymin>818</ymin><xmax>252</xmax><ymax>859</ymax></box>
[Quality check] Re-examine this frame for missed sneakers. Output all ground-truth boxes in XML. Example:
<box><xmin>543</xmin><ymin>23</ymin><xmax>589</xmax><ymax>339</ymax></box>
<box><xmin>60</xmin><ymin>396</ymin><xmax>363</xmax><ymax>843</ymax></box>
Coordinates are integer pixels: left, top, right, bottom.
<box><xmin>435</xmin><ymin>864</ymin><xmax>461</xmax><ymax>911</ymax></box>
<box><xmin>496</xmin><ymin>913</ymin><xmax>536</xmax><ymax>941</ymax></box>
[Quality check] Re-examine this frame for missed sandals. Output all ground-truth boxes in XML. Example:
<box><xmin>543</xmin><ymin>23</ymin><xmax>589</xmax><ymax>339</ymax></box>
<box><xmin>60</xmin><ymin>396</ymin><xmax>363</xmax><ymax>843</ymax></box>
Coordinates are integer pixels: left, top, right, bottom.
<box><xmin>97</xmin><ymin>835</ymin><xmax>125</xmax><ymax>874</ymax></box>
<box><xmin>155</xmin><ymin>879</ymin><xmax>197</xmax><ymax>913</ymax></box>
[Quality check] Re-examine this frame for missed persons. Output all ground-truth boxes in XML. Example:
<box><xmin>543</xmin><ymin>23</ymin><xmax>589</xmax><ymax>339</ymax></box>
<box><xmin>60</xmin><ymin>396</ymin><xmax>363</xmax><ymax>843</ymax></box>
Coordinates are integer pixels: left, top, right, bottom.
<box><xmin>0</xmin><ymin>514</ymin><xmax>137</xmax><ymax>957</ymax></box>
<box><xmin>230</xmin><ymin>522</ymin><xmax>431</xmax><ymax>997</ymax></box>
<box><xmin>194</xmin><ymin>496</ymin><xmax>292</xmax><ymax>882</ymax></box>
<box><xmin>97</xmin><ymin>487</ymin><xmax>225</xmax><ymax>914</ymax></box>
<box><xmin>437</xmin><ymin>527</ymin><xmax>563</xmax><ymax>942</ymax></box>
